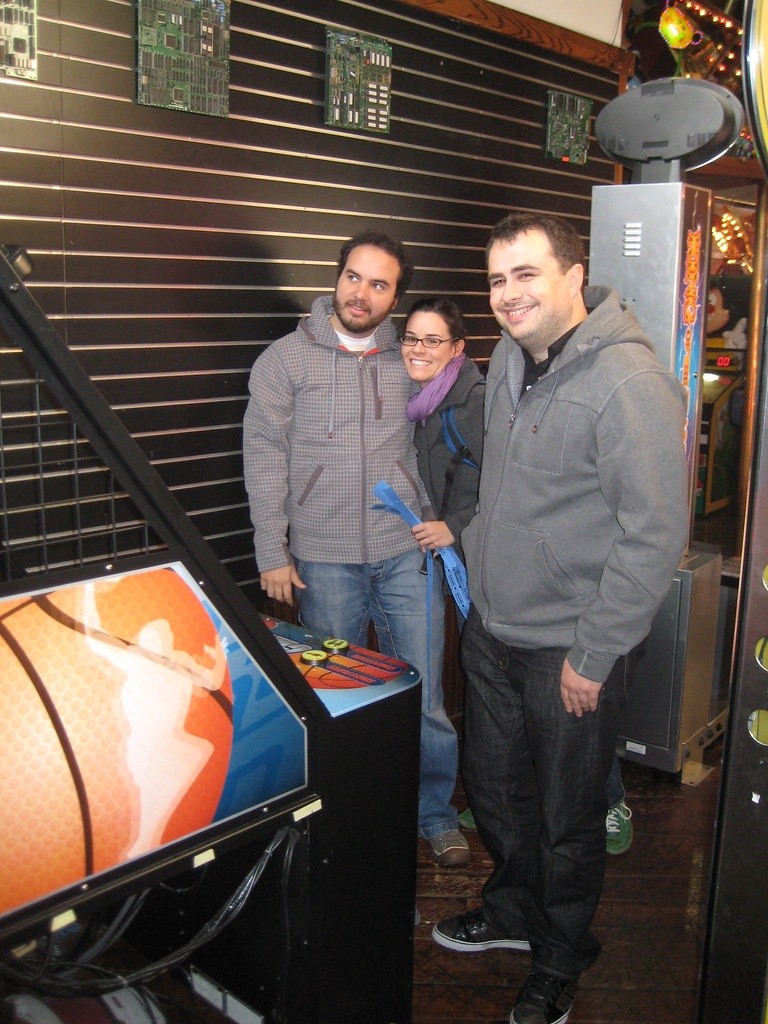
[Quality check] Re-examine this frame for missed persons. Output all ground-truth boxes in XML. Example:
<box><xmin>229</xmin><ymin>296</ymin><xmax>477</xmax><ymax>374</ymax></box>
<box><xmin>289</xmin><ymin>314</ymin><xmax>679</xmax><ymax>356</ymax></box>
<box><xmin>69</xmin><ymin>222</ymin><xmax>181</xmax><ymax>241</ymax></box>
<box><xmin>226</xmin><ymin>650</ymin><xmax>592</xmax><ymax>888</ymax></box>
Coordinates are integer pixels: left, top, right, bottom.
<box><xmin>432</xmin><ymin>215</ymin><xmax>685</xmax><ymax>1023</ymax></box>
<box><xmin>243</xmin><ymin>232</ymin><xmax>470</xmax><ymax>867</ymax></box>
<box><xmin>400</xmin><ymin>299</ymin><xmax>633</xmax><ymax>854</ymax></box>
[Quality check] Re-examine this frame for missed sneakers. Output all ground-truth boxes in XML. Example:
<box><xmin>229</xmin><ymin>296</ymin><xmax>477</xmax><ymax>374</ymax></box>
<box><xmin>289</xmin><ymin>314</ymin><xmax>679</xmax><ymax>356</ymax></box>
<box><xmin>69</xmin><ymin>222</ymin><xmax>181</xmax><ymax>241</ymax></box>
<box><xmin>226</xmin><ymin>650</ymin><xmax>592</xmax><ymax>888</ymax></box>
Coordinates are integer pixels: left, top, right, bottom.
<box><xmin>509</xmin><ymin>964</ymin><xmax>579</xmax><ymax>1024</ymax></box>
<box><xmin>430</xmin><ymin>830</ymin><xmax>471</xmax><ymax>866</ymax></box>
<box><xmin>604</xmin><ymin>799</ymin><xmax>634</xmax><ymax>855</ymax></box>
<box><xmin>432</xmin><ymin>907</ymin><xmax>532</xmax><ymax>951</ymax></box>
<box><xmin>458</xmin><ymin>807</ymin><xmax>477</xmax><ymax>829</ymax></box>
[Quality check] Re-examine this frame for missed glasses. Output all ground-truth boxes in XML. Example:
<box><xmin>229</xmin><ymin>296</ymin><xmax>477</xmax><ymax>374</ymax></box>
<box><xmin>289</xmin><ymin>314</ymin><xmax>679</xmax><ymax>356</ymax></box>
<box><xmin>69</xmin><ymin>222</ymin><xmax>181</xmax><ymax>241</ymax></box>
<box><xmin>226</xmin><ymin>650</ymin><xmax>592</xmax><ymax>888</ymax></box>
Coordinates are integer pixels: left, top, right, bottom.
<box><xmin>397</xmin><ymin>335</ymin><xmax>458</xmax><ymax>348</ymax></box>
